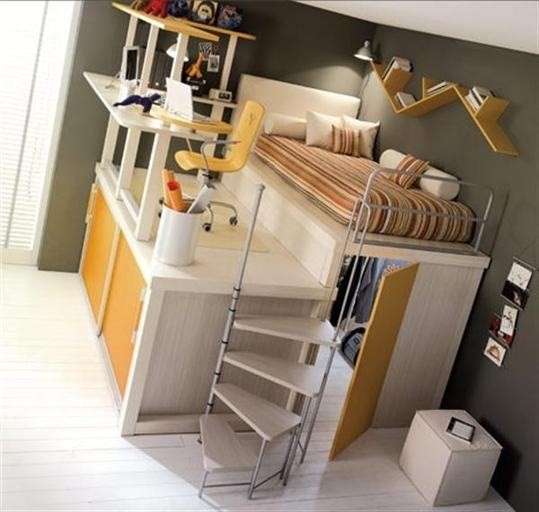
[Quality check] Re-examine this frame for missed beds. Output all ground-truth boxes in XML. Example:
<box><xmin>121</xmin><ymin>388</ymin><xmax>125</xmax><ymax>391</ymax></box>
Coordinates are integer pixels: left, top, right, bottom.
<box><xmin>218</xmin><ymin>74</ymin><xmax>491</xmax><ymax>286</ymax></box>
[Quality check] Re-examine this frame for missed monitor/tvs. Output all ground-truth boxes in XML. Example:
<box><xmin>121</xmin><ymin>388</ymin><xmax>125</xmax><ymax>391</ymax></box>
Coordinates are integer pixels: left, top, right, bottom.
<box><xmin>119</xmin><ymin>45</ymin><xmax>141</xmax><ymax>90</ymax></box>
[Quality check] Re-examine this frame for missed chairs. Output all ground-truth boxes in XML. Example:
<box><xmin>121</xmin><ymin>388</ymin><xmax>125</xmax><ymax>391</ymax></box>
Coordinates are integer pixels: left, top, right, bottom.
<box><xmin>158</xmin><ymin>99</ymin><xmax>265</xmax><ymax>232</ymax></box>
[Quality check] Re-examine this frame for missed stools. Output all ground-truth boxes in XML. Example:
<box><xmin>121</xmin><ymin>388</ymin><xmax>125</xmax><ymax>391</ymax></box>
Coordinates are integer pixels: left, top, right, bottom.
<box><xmin>398</xmin><ymin>408</ymin><xmax>504</xmax><ymax>507</ymax></box>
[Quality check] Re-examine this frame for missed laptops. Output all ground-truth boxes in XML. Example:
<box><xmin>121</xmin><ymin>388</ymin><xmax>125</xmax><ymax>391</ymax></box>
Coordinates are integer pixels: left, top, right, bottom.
<box><xmin>166</xmin><ymin>77</ymin><xmax>212</xmax><ymax>122</ymax></box>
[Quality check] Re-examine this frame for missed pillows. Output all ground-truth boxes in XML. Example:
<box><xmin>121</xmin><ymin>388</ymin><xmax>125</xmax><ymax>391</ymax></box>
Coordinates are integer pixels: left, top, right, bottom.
<box><xmin>388</xmin><ymin>154</ymin><xmax>430</xmax><ymax>190</ymax></box>
<box><xmin>330</xmin><ymin>123</ymin><xmax>360</xmax><ymax>158</ymax></box>
<box><xmin>265</xmin><ymin>112</ymin><xmax>307</xmax><ymax>140</ymax></box>
<box><xmin>343</xmin><ymin>115</ymin><xmax>380</xmax><ymax>160</ymax></box>
<box><xmin>379</xmin><ymin>148</ymin><xmax>459</xmax><ymax>201</ymax></box>
<box><xmin>305</xmin><ymin>109</ymin><xmax>342</xmax><ymax>146</ymax></box>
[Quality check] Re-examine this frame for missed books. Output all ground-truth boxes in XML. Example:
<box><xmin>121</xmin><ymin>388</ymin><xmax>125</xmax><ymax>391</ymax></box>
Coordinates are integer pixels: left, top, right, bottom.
<box><xmin>382</xmin><ymin>56</ymin><xmax>496</xmax><ymax>115</ymax></box>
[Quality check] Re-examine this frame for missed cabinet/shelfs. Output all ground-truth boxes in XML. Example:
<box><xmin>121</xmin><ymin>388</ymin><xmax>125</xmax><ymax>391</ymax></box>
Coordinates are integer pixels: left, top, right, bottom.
<box><xmin>370</xmin><ymin>61</ymin><xmax>520</xmax><ymax>156</ymax></box>
<box><xmin>82</xmin><ymin>1</ymin><xmax>259</xmax><ymax>243</ymax></box>
<box><xmin>97</xmin><ymin>229</ymin><xmax>326</xmax><ymax>442</ymax></box>
<box><xmin>79</xmin><ymin>167</ymin><xmax>286</xmax><ymax>327</ymax></box>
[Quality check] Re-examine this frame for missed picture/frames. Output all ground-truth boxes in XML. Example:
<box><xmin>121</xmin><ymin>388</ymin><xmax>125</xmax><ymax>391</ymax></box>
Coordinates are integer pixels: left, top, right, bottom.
<box><xmin>481</xmin><ymin>260</ymin><xmax>534</xmax><ymax>369</ymax></box>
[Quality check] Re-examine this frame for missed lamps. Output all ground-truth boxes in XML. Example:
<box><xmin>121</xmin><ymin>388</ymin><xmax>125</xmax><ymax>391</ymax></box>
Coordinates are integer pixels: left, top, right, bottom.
<box><xmin>353</xmin><ymin>40</ymin><xmax>378</xmax><ymax>63</ymax></box>
